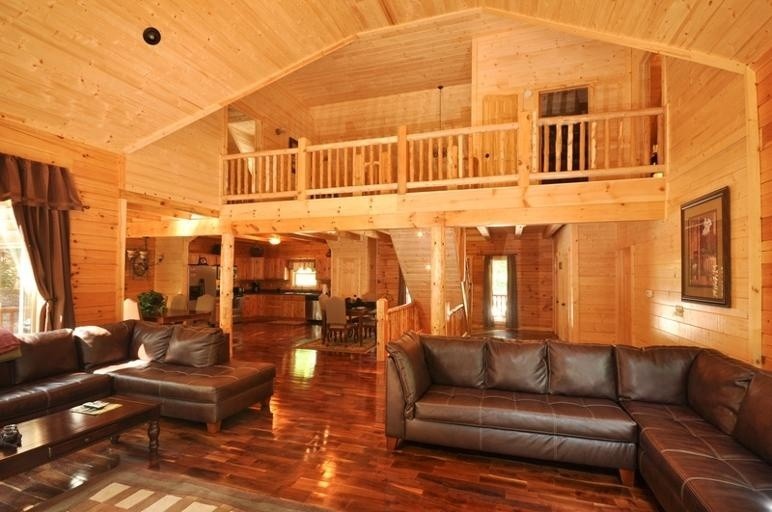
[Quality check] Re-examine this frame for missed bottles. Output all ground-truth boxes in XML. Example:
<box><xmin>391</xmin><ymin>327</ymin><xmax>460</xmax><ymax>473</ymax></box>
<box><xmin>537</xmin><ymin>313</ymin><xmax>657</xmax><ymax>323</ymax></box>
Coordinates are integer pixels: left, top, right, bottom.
<box><xmin>4</xmin><ymin>424</ymin><xmax>19</xmax><ymax>443</ymax></box>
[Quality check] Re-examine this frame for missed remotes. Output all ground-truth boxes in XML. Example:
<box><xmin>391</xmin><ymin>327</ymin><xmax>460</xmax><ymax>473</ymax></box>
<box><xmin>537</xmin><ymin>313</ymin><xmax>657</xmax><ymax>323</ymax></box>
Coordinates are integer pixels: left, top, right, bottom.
<box><xmin>83</xmin><ymin>402</ymin><xmax>105</xmax><ymax>409</ymax></box>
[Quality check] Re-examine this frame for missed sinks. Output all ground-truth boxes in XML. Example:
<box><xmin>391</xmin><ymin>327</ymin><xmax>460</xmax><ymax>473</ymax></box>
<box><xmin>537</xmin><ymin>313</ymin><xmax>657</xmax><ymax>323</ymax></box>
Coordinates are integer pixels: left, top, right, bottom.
<box><xmin>281</xmin><ymin>292</ymin><xmax>313</xmax><ymax>295</ymax></box>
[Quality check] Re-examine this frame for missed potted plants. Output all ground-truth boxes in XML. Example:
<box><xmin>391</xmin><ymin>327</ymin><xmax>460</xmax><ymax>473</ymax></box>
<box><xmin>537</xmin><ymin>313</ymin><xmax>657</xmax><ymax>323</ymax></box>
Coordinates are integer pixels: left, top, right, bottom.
<box><xmin>137</xmin><ymin>290</ymin><xmax>166</xmax><ymax>315</ymax></box>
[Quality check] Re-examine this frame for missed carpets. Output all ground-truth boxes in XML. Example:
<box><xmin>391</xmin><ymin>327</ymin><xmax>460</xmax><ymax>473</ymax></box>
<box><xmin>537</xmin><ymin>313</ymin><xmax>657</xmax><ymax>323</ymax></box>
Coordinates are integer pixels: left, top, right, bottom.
<box><xmin>20</xmin><ymin>461</ymin><xmax>335</xmax><ymax>511</ymax></box>
<box><xmin>293</xmin><ymin>338</ymin><xmax>378</xmax><ymax>355</ymax></box>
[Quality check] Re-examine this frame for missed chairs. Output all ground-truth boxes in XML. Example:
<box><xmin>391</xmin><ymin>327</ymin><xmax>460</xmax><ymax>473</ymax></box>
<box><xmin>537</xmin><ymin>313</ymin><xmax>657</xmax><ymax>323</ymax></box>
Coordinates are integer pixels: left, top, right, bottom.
<box><xmin>324</xmin><ymin>296</ymin><xmax>359</xmax><ymax>347</ymax></box>
<box><xmin>124</xmin><ymin>298</ymin><xmax>143</xmax><ymax>321</ymax></box>
<box><xmin>196</xmin><ymin>294</ymin><xmax>216</xmax><ymax>324</ymax></box>
<box><xmin>170</xmin><ymin>293</ymin><xmax>189</xmax><ymax>310</ymax></box>
<box><xmin>318</xmin><ymin>294</ymin><xmax>331</xmax><ymax>347</ymax></box>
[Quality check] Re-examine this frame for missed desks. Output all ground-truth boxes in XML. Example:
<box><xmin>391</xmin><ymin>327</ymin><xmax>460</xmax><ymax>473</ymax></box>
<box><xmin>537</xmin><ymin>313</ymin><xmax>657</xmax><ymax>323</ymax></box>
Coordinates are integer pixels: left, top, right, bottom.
<box><xmin>141</xmin><ymin>308</ymin><xmax>212</xmax><ymax>325</ymax></box>
<box><xmin>345</xmin><ymin>299</ymin><xmax>378</xmax><ymax>346</ymax></box>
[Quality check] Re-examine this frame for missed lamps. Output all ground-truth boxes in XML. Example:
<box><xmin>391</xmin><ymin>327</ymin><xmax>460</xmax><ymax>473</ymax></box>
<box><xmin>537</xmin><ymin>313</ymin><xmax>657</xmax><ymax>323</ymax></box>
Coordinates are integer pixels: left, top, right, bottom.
<box><xmin>127</xmin><ymin>237</ymin><xmax>164</xmax><ymax>268</ymax></box>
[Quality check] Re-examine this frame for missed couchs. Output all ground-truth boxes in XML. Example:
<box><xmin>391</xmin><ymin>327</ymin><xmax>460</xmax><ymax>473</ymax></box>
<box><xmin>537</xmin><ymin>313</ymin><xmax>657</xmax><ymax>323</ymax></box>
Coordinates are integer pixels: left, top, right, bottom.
<box><xmin>1</xmin><ymin>321</ymin><xmax>275</xmax><ymax>434</ymax></box>
<box><xmin>385</xmin><ymin>329</ymin><xmax>772</xmax><ymax>510</ymax></box>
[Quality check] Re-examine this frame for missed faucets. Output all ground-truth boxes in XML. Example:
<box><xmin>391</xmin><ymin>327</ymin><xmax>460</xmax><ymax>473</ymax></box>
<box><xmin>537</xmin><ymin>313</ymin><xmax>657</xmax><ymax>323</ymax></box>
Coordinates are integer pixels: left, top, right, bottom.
<box><xmin>301</xmin><ymin>285</ymin><xmax>303</xmax><ymax>292</ymax></box>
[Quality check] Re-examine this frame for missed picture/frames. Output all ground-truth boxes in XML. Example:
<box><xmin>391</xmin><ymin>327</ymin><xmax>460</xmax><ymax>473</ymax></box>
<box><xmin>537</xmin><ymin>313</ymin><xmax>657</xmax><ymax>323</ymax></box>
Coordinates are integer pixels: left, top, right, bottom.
<box><xmin>679</xmin><ymin>187</ymin><xmax>732</xmax><ymax>310</ymax></box>
